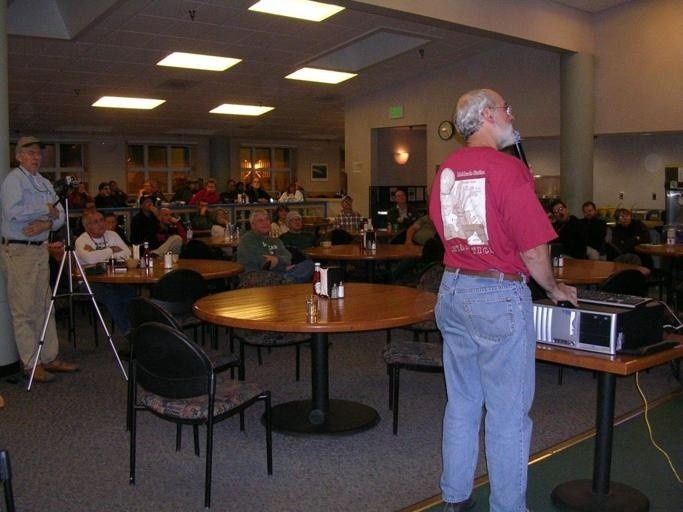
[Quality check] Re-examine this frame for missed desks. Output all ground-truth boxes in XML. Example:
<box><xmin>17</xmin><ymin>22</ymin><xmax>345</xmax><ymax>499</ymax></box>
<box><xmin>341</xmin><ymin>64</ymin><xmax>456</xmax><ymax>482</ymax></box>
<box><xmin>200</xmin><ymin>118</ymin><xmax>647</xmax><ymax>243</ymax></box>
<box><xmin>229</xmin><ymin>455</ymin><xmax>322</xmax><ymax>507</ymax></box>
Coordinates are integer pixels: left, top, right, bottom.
<box><xmin>68</xmin><ymin>259</ymin><xmax>245</xmax><ymax>348</ymax></box>
<box><xmin>192</xmin><ymin>280</ymin><xmax>440</xmax><ymax>437</ymax></box>
<box><xmin>536</xmin><ymin>333</ymin><xmax>683</xmax><ymax>510</ymax></box>
<box><xmin>194</xmin><ymin>236</ymin><xmax>239</xmax><ymax>257</ymax></box>
<box><xmin>302</xmin><ymin>229</ymin><xmax>423</xmax><ymax>282</ymax></box>
<box><xmin>551</xmin><ymin>242</ymin><xmax>681</xmax><ymax>289</ymax></box>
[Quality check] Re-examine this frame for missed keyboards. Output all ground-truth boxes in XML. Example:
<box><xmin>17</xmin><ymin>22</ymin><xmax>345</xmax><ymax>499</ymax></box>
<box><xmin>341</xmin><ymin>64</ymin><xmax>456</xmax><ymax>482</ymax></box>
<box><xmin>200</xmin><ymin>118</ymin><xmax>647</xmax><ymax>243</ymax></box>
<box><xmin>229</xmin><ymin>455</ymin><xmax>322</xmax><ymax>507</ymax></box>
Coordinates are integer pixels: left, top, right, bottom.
<box><xmin>575</xmin><ymin>285</ymin><xmax>655</xmax><ymax>310</ymax></box>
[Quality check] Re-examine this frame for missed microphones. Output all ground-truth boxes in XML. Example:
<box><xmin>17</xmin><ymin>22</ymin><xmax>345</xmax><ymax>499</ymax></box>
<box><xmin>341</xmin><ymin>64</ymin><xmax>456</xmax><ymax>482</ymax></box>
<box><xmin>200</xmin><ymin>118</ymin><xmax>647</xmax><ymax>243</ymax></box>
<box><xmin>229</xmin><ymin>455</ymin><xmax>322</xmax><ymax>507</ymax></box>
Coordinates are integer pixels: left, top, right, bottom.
<box><xmin>512</xmin><ymin>130</ymin><xmax>531</xmax><ymax>166</ymax></box>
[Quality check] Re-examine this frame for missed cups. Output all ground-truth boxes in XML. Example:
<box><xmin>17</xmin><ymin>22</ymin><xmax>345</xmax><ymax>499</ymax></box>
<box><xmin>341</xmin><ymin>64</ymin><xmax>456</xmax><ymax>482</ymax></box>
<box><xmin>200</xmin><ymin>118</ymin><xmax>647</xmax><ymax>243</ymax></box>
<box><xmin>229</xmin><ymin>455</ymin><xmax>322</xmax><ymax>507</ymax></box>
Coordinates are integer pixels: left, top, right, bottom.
<box><xmin>319</xmin><ymin>241</ymin><xmax>331</xmax><ymax>248</ymax></box>
<box><xmin>305</xmin><ymin>296</ymin><xmax>318</xmax><ymax>316</ymax></box>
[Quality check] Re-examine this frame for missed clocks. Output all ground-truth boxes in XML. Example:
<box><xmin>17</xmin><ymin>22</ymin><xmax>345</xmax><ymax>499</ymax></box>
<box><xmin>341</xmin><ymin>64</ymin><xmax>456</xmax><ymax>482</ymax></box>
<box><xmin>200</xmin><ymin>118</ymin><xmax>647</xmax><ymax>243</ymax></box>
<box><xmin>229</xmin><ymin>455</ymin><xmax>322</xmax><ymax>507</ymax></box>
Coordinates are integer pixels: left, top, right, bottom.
<box><xmin>438</xmin><ymin>120</ymin><xmax>453</xmax><ymax>140</ymax></box>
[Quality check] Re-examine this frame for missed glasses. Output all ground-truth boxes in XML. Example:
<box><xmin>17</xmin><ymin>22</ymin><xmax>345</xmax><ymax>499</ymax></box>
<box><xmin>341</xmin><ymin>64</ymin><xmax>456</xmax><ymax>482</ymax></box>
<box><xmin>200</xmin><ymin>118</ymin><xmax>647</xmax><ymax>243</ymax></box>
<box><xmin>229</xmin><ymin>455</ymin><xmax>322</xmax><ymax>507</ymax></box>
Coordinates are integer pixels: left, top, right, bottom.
<box><xmin>488</xmin><ymin>104</ymin><xmax>512</xmax><ymax>115</ymax></box>
<box><xmin>554</xmin><ymin>208</ymin><xmax>563</xmax><ymax>213</ymax></box>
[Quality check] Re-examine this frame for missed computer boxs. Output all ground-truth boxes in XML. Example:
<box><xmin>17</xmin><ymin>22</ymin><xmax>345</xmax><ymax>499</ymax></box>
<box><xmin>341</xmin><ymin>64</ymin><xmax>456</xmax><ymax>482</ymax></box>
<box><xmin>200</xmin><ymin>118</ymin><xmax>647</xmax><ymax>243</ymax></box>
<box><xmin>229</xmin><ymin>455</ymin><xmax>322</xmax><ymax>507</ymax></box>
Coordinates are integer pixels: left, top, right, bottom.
<box><xmin>531</xmin><ymin>296</ymin><xmax>664</xmax><ymax>356</ymax></box>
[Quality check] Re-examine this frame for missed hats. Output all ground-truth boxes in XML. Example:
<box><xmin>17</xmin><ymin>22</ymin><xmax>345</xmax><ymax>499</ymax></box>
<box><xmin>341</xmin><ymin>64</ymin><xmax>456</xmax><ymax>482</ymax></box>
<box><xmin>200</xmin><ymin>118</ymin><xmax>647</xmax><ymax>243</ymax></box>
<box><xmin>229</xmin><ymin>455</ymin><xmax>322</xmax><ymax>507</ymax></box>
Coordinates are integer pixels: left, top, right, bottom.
<box><xmin>17</xmin><ymin>137</ymin><xmax>40</xmax><ymax>147</ymax></box>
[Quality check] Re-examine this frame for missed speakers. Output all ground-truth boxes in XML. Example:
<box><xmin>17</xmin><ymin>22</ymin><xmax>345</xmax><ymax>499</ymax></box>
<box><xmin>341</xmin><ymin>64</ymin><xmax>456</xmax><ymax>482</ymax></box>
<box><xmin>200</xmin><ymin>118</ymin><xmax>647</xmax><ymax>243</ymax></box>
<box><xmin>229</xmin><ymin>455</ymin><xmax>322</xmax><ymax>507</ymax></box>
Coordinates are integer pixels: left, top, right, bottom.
<box><xmin>664</xmin><ymin>166</ymin><xmax>679</xmax><ymax>190</ymax></box>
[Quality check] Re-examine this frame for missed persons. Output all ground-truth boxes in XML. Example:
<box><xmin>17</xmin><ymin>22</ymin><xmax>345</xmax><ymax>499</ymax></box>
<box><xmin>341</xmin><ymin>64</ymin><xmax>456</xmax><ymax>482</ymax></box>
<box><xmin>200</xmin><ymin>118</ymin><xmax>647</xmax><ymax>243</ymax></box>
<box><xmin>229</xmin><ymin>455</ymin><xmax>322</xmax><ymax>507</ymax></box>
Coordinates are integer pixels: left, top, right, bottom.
<box><xmin>330</xmin><ymin>190</ymin><xmax>440</xmax><ymax>290</ymax></box>
<box><xmin>428</xmin><ymin>87</ymin><xmax>580</xmax><ymax>512</ymax></box>
<box><xmin>1</xmin><ymin>135</ymin><xmax>79</xmax><ymax>381</ymax></box>
<box><xmin>534</xmin><ymin>188</ymin><xmax>657</xmax><ymax>277</ymax></box>
<box><xmin>47</xmin><ymin>175</ymin><xmax>314</xmax><ymax>290</ymax></box>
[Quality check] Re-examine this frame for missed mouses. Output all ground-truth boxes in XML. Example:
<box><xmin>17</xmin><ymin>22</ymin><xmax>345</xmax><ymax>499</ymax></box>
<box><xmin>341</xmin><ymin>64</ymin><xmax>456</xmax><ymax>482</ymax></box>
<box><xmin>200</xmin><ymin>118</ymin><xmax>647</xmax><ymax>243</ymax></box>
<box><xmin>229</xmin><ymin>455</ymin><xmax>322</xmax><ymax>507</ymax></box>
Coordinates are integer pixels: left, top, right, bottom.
<box><xmin>558</xmin><ymin>298</ymin><xmax>574</xmax><ymax>309</ymax></box>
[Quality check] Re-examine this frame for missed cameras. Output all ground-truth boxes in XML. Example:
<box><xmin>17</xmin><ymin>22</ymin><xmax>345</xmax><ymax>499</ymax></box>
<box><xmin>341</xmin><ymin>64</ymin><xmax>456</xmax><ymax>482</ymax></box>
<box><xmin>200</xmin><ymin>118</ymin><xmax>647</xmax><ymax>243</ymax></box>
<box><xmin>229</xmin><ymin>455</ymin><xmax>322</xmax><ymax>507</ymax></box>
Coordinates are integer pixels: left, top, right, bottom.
<box><xmin>52</xmin><ymin>173</ymin><xmax>77</xmax><ymax>198</ymax></box>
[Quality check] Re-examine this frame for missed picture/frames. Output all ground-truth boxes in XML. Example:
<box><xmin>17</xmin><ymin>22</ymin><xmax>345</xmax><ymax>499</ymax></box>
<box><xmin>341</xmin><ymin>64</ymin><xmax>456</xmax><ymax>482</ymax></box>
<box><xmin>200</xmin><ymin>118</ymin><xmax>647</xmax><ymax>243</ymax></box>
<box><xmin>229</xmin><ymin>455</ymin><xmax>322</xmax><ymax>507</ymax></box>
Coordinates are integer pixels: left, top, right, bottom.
<box><xmin>310</xmin><ymin>163</ymin><xmax>329</xmax><ymax>181</ymax></box>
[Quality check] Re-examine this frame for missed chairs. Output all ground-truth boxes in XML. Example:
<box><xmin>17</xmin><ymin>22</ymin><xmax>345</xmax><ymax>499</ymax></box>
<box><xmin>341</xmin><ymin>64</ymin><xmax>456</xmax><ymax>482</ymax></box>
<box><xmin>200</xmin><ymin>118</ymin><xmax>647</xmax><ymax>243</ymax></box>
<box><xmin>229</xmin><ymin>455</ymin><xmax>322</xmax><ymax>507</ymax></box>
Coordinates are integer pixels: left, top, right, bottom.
<box><xmin>604</xmin><ymin>269</ymin><xmax>647</xmax><ymax>298</ymax></box>
<box><xmin>384</xmin><ymin>259</ymin><xmax>448</xmax><ymax>434</ymax></box>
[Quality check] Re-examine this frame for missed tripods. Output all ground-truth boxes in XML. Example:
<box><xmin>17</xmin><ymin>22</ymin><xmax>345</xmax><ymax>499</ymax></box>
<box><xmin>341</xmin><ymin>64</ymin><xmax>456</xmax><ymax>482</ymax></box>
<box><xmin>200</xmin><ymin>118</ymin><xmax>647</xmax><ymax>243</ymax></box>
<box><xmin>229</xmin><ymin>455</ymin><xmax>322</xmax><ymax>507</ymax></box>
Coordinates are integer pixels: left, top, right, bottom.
<box><xmin>26</xmin><ymin>200</ymin><xmax>130</xmax><ymax>393</ymax></box>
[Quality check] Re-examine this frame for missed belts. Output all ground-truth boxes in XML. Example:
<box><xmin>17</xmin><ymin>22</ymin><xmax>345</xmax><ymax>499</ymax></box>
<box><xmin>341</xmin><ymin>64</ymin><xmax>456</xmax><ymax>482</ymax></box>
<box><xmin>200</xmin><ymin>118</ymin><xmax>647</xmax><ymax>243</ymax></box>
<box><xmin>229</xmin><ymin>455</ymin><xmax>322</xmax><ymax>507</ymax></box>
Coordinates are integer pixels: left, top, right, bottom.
<box><xmin>445</xmin><ymin>265</ymin><xmax>523</xmax><ymax>283</ymax></box>
<box><xmin>2</xmin><ymin>237</ymin><xmax>43</xmax><ymax>246</ymax></box>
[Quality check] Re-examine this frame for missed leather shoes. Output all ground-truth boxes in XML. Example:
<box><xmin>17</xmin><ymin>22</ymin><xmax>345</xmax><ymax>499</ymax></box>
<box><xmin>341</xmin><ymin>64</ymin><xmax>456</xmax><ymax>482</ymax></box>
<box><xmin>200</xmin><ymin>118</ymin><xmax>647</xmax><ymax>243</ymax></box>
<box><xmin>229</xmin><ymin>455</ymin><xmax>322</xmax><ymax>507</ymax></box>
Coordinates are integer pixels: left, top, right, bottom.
<box><xmin>442</xmin><ymin>492</ymin><xmax>475</xmax><ymax>512</ymax></box>
<box><xmin>24</xmin><ymin>365</ymin><xmax>55</xmax><ymax>382</ymax></box>
<box><xmin>43</xmin><ymin>356</ymin><xmax>79</xmax><ymax>371</ymax></box>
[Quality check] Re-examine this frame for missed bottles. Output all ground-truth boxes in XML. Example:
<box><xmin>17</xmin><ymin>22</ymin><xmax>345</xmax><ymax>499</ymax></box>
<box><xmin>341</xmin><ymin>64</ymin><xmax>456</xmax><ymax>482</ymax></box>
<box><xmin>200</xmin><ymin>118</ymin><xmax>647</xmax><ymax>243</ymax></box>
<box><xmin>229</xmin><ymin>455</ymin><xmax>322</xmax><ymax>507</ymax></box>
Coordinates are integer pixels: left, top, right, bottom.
<box><xmin>386</xmin><ymin>222</ymin><xmax>392</xmax><ymax>234</ymax></box>
<box><xmin>330</xmin><ymin>281</ymin><xmax>344</xmax><ymax>299</ymax></box>
<box><xmin>139</xmin><ymin>242</ymin><xmax>153</xmax><ymax>268</ymax></box>
<box><xmin>223</xmin><ymin>225</ymin><xmax>240</xmax><ymax>241</ymax></box>
<box><xmin>108</xmin><ymin>254</ymin><xmax>115</xmax><ymax>274</ymax></box>
<box><xmin>359</xmin><ymin>228</ymin><xmax>376</xmax><ymax>250</ymax></box>
<box><xmin>666</xmin><ymin>229</ymin><xmax>675</xmax><ymax>246</ymax></box>
<box><xmin>240</xmin><ymin>193</ymin><xmax>245</xmax><ymax>206</ymax></box>
<box><xmin>311</xmin><ymin>261</ymin><xmax>321</xmax><ymax>297</ymax></box>
<box><xmin>163</xmin><ymin>252</ymin><xmax>172</xmax><ymax>269</ymax></box>
<box><xmin>551</xmin><ymin>254</ymin><xmax>564</xmax><ymax>267</ymax></box>
